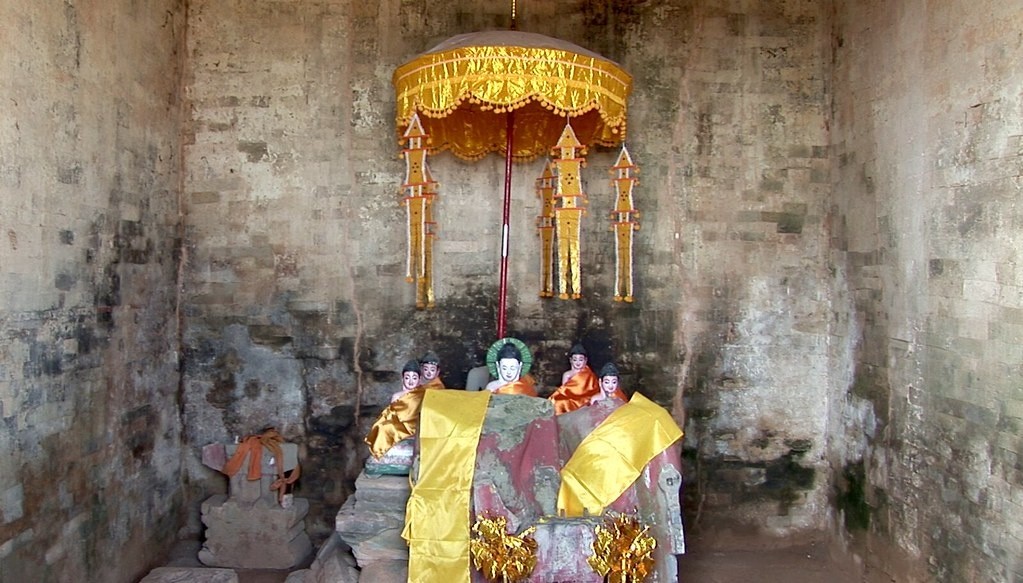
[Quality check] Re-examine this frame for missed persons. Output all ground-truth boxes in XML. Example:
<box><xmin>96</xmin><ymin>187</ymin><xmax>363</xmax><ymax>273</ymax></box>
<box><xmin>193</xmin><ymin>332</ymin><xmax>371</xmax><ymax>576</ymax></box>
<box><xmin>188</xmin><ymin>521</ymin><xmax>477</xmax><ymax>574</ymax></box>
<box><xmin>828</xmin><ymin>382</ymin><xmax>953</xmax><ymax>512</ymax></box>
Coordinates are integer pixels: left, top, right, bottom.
<box><xmin>390</xmin><ymin>358</ymin><xmax>424</xmax><ymax>437</ymax></box>
<box><xmin>590</xmin><ymin>362</ymin><xmax>628</xmax><ymax>405</ymax></box>
<box><xmin>547</xmin><ymin>343</ymin><xmax>599</xmax><ymax>416</ymax></box>
<box><xmin>486</xmin><ymin>342</ymin><xmax>538</xmax><ymax>398</ymax></box>
<box><xmin>420</xmin><ymin>350</ymin><xmax>446</xmax><ymax>389</ymax></box>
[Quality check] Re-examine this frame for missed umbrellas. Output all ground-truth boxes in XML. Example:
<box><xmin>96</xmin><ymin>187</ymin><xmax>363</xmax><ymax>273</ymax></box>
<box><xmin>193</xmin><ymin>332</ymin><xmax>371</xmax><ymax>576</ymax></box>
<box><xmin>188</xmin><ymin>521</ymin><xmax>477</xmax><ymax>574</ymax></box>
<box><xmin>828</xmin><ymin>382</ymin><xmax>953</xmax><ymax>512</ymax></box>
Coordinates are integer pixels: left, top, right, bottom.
<box><xmin>390</xmin><ymin>0</ymin><xmax>641</xmax><ymax>342</ymax></box>
